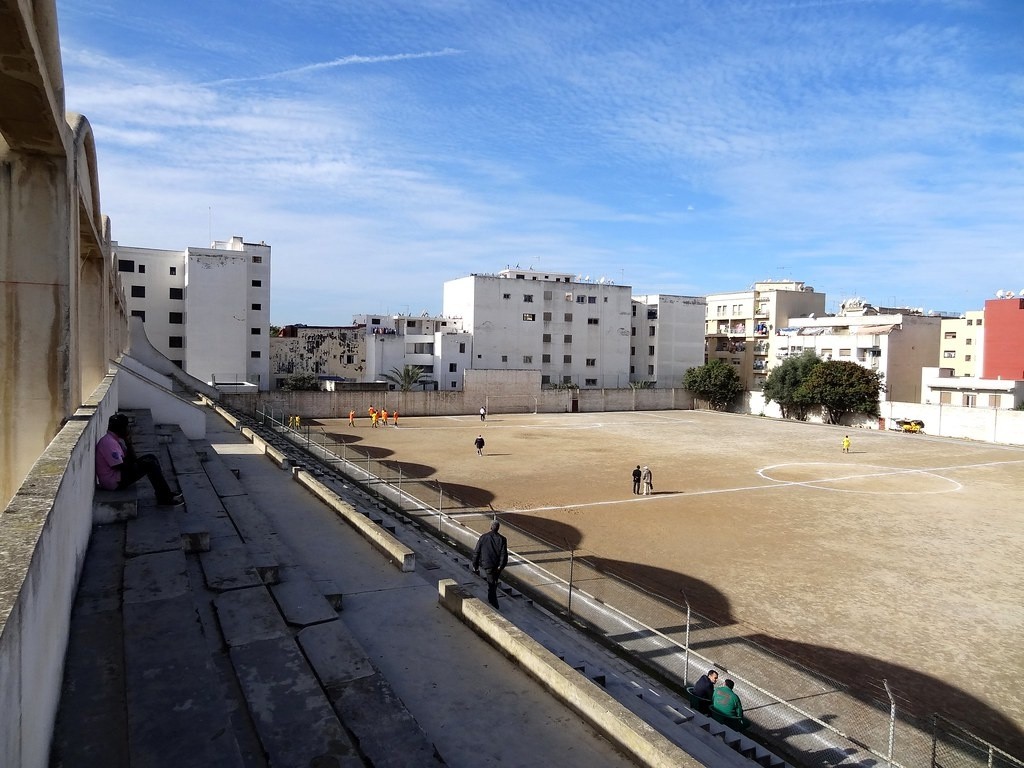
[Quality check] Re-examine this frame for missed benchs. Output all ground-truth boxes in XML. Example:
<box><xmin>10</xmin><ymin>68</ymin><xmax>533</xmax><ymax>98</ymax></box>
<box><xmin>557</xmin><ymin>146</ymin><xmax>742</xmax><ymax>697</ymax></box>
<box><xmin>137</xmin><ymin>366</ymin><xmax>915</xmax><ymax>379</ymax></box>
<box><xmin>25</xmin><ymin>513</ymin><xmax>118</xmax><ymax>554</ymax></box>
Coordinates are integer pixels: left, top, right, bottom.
<box><xmin>94</xmin><ymin>480</ymin><xmax>138</xmax><ymax>519</ymax></box>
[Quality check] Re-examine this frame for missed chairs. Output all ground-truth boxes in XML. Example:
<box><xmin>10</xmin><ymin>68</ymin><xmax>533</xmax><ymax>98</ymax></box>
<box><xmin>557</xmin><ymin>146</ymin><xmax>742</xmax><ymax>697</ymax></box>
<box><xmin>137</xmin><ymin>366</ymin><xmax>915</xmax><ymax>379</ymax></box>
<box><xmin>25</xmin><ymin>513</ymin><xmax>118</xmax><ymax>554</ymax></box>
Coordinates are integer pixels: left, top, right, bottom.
<box><xmin>685</xmin><ymin>686</ymin><xmax>712</xmax><ymax>714</ymax></box>
<box><xmin>707</xmin><ymin>704</ymin><xmax>742</xmax><ymax>731</ymax></box>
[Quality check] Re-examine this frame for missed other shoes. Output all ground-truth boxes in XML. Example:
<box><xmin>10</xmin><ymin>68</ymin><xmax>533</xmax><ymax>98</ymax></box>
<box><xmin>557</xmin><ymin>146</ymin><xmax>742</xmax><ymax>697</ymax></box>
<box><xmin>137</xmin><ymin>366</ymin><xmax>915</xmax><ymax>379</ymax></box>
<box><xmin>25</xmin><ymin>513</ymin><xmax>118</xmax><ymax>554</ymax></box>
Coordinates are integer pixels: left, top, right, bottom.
<box><xmin>643</xmin><ymin>492</ymin><xmax>647</xmax><ymax>495</ymax></box>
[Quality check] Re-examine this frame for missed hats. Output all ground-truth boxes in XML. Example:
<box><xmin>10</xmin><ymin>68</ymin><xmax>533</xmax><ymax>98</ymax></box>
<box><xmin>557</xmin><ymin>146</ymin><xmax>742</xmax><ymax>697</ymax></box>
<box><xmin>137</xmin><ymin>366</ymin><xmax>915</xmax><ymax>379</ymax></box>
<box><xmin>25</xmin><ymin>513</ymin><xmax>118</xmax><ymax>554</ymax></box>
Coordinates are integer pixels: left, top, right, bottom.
<box><xmin>643</xmin><ymin>466</ymin><xmax>648</xmax><ymax>470</ymax></box>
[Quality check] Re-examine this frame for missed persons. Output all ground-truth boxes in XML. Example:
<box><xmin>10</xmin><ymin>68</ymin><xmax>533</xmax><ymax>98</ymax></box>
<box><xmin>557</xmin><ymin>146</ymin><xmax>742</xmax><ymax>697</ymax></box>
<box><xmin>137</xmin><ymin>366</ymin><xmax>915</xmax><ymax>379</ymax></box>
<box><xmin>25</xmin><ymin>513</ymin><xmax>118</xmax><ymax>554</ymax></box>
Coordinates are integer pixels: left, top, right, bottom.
<box><xmin>374</xmin><ymin>327</ymin><xmax>396</xmax><ymax>334</ymax></box>
<box><xmin>95</xmin><ymin>414</ymin><xmax>185</xmax><ymax>508</ymax></box>
<box><xmin>842</xmin><ymin>436</ymin><xmax>851</xmax><ymax>453</ymax></box>
<box><xmin>631</xmin><ymin>465</ymin><xmax>642</xmax><ymax>496</ymax></box>
<box><xmin>287</xmin><ymin>414</ymin><xmax>294</xmax><ymax>432</ymax></box>
<box><xmin>642</xmin><ymin>466</ymin><xmax>653</xmax><ymax>495</ymax></box>
<box><xmin>474</xmin><ymin>435</ymin><xmax>485</xmax><ymax>456</ymax></box>
<box><xmin>480</xmin><ymin>406</ymin><xmax>486</xmax><ymax>421</ymax></box>
<box><xmin>394</xmin><ymin>410</ymin><xmax>398</xmax><ymax>428</ymax></box>
<box><xmin>348</xmin><ymin>409</ymin><xmax>356</xmax><ymax>427</ymax></box>
<box><xmin>372</xmin><ymin>409</ymin><xmax>380</xmax><ymax>428</ymax></box>
<box><xmin>368</xmin><ymin>405</ymin><xmax>374</xmax><ymax>420</ymax></box>
<box><xmin>382</xmin><ymin>407</ymin><xmax>388</xmax><ymax>428</ymax></box>
<box><xmin>691</xmin><ymin>670</ymin><xmax>744</xmax><ymax>731</ymax></box>
<box><xmin>295</xmin><ymin>414</ymin><xmax>302</xmax><ymax>430</ymax></box>
<box><xmin>472</xmin><ymin>522</ymin><xmax>508</xmax><ymax>610</ymax></box>
